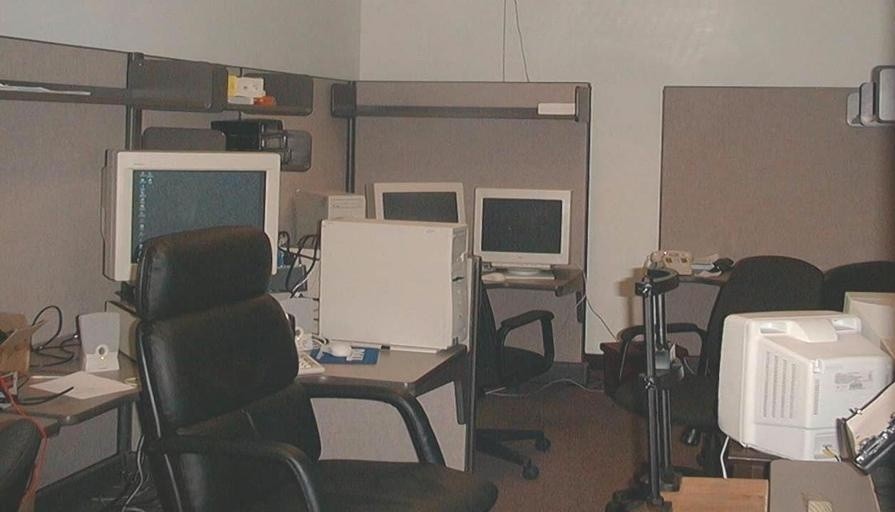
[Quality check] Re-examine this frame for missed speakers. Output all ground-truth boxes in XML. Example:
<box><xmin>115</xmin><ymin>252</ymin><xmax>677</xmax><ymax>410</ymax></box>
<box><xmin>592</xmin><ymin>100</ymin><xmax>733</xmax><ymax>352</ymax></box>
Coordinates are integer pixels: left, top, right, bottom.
<box><xmin>278</xmin><ymin>297</ymin><xmax>313</xmax><ymax>352</ymax></box>
<box><xmin>78</xmin><ymin>311</ymin><xmax>121</xmax><ymax>373</ymax></box>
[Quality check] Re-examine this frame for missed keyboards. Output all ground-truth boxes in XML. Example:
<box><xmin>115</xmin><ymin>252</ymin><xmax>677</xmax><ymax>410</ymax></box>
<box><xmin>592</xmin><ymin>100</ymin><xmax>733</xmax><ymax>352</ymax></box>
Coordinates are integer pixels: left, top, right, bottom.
<box><xmin>297</xmin><ymin>351</ymin><xmax>326</xmax><ymax>375</ymax></box>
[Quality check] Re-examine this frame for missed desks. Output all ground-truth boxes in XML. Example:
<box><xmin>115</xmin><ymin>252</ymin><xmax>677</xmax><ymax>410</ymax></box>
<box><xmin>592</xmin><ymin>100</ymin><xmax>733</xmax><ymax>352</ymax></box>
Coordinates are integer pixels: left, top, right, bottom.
<box><xmin>482</xmin><ymin>262</ymin><xmax>585</xmax><ymax>323</ymax></box>
<box><xmin>0</xmin><ymin>331</ymin><xmax>471</xmax><ymax>483</ymax></box>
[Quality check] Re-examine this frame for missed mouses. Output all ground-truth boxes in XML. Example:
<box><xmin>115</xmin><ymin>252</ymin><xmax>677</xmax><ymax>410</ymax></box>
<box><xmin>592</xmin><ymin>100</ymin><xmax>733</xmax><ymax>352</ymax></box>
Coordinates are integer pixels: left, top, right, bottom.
<box><xmin>320</xmin><ymin>341</ymin><xmax>352</xmax><ymax>358</ymax></box>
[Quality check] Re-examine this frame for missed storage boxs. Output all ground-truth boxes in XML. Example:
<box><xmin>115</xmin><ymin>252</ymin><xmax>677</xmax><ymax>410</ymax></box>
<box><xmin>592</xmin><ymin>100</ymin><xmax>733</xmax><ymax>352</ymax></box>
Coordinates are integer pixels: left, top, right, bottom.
<box><xmin>0</xmin><ymin>310</ymin><xmax>47</xmax><ymax>376</ymax></box>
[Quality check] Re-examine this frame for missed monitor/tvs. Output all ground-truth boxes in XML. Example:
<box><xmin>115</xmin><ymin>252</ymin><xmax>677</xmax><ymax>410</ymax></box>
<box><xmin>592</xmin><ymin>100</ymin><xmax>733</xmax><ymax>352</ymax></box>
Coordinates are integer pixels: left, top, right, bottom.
<box><xmin>843</xmin><ymin>291</ymin><xmax>895</xmax><ymax>365</ymax></box>
<box><xmin>365</xmin><ymin>181</ymin><xmax>466</xmax><ymax>224</ymax></box>
<box><xmin>100</xmin><ymin>149</ymin><xmax>281</xmax><ymax>282</ymax></box>
<box><xmin>715</xmin><ymin>310</ymin><xmax>895</xmax><ymax>463</ymax></box>
<box><xmin>472</xmin><ymin>187</ymin><xmax>572</xmax><ymax>280</ymax></box>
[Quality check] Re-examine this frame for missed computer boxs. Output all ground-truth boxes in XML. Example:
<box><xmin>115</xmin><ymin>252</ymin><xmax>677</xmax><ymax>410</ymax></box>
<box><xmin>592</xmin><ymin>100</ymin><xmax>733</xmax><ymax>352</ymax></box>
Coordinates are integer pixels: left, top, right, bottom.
<box><xmin>318</xmin><ymin>216</ymin><xmax>469</xmax><ymax>354</ymax></box>
<box><xmin>294</xmin><ymin>189</ymin><xmax>366</xmax><ymax>248</ymax></box>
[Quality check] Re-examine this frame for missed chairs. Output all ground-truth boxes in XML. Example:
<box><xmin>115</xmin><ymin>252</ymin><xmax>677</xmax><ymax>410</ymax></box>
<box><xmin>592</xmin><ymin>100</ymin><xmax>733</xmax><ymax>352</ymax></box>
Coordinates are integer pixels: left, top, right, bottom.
<box><xmin>468</xmin><ymin>253</ymin><xmax>555</xmax><ymax>482</ymax></box>
<box><xmin>128</xmin><ymin>224</ymin><xmax>498</xmax><ymax>512</ymax></box>
<box><xmin>606</xmin><ymin>256</ymin><xmax>895</xmax><ymax>511</ymax></box>
